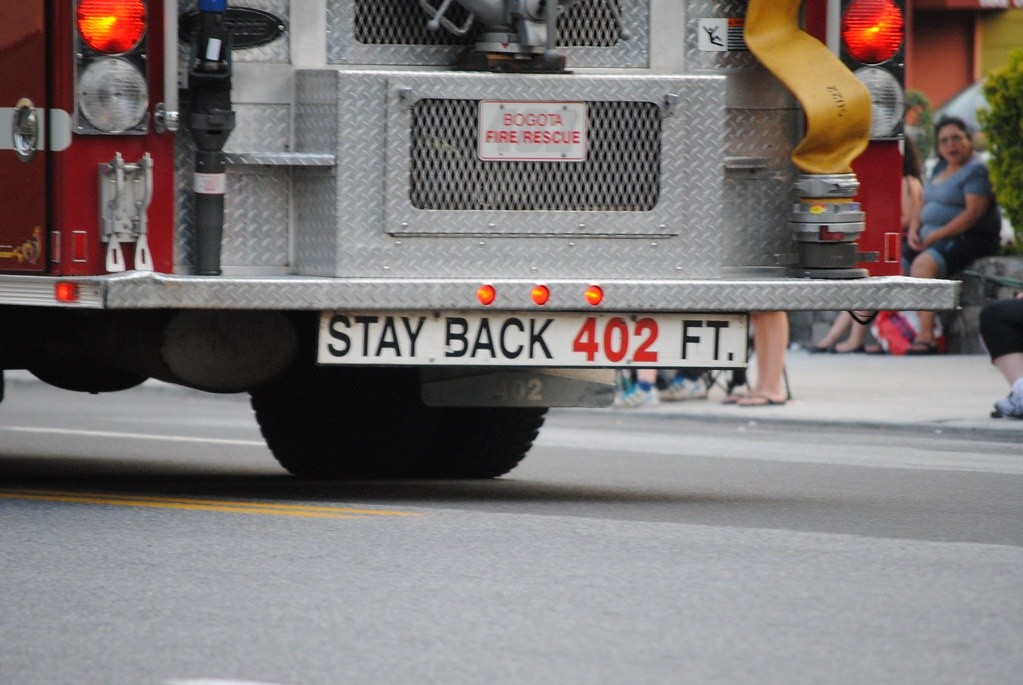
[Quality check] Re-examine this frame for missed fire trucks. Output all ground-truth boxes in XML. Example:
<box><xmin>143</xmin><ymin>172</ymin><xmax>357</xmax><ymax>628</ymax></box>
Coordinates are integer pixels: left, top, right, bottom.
<box><xmin>0</xmin><ymin>0</ymin><xmax>963</xmax><ymax>482</ymax></box>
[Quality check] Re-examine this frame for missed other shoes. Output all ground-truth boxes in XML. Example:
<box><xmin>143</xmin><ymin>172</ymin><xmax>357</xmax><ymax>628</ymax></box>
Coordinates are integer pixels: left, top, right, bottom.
<box><xmin>829</xmin><ymin>342</ymin><xmax>866</xmax><ymax>354</ymax></box>
<box><xmin>803</xmin><ymin>342</ymin><xmax>835</xmax><ymax>352</ymax></box>
<box><xmin>993</xmin><ymin>389</ymin><xmax>1023</xmax><ymax>417</ymax></box>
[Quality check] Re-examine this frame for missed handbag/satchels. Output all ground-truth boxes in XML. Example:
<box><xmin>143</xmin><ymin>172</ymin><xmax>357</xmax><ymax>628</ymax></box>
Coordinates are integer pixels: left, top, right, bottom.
<box><xmin>870</xmin><ymin>311</ymin><xmax>947</xmax><ymax>356</ymax></box>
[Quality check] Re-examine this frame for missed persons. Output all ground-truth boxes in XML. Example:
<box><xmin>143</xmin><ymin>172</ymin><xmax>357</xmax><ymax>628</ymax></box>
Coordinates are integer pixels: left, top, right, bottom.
<box><xmin>607</xmin><ymin>310</ymin><xmax>789</xmax><ymax>410</ymax></box>
<box><xmin>977</xmin><ymin>288</ymin><xmax>1023</xmax><ymax>421</ymax></box>
<box><xmin>861</xmin><ymin>116</ymin><xmax>1004</xmax><ymax>356</ymax></box>
<box><xmin>805</xmin><ymin>124</ymin><xmax>926</xmax><ymax>356</ymax></box>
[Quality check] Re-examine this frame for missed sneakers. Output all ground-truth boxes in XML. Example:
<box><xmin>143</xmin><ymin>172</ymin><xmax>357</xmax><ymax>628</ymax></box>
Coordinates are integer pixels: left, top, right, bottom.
<box><xmin>658</xmin><ymin>376</ymin><xmax>708</xmax><ymax>401</ymax></box>
<box><xmin>614</xmin><ymin>384</ymin><xmax>658</xmax><ymax>409</ymax></box>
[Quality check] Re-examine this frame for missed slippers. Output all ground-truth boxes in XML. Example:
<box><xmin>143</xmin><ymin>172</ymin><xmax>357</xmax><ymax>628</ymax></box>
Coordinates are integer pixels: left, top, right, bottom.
<box><xmin>722</xmin><ymin>392</ymin><xmax>744</xmax><ymax>404</ymax></box>
<box><xmin>906</xmin><ymin>340</ymin><xmax>939</xmax><ymax>354</ymax></box>
<box><xmin>737</xmin><ymin>395</ymin><xmax>785</xmax><ymax>407</ymax></box>
<box><xmin>863</xmin><ymin>342</ymin><xmax>891</xmax><ymax>354</ymax></box>
